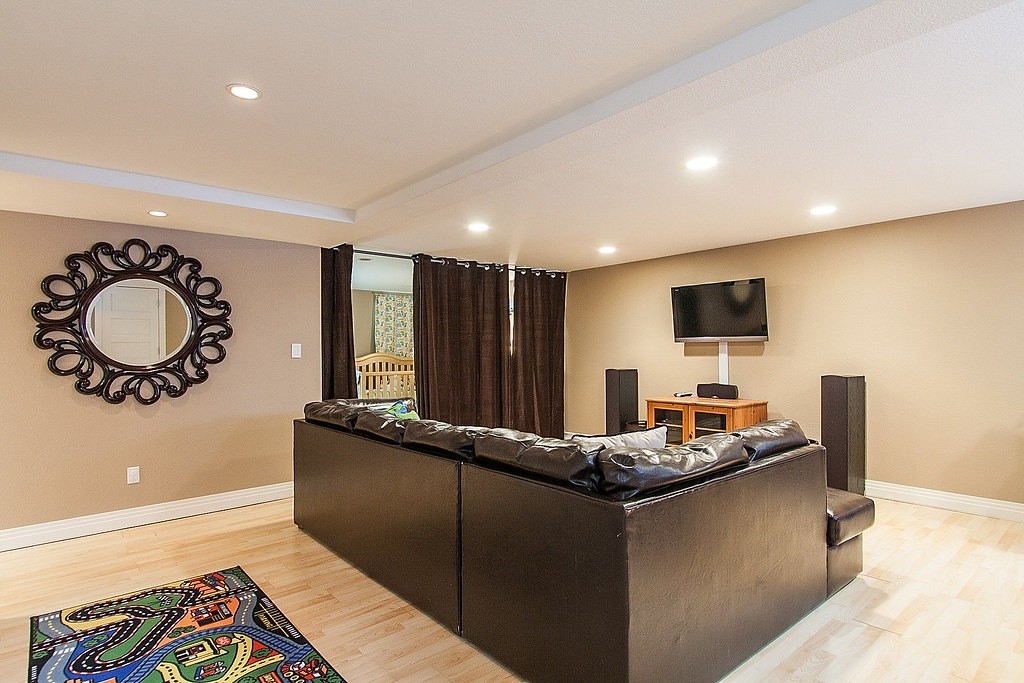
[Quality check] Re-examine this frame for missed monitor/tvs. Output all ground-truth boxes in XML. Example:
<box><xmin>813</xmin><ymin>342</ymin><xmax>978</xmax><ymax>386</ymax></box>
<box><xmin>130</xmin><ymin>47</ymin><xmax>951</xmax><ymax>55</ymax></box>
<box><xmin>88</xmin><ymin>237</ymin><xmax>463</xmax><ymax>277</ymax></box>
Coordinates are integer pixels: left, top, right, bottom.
<box><xmin>670</xmin><ymin>277</ymin><xmax>768</xmax><ymax>342</ymax></box>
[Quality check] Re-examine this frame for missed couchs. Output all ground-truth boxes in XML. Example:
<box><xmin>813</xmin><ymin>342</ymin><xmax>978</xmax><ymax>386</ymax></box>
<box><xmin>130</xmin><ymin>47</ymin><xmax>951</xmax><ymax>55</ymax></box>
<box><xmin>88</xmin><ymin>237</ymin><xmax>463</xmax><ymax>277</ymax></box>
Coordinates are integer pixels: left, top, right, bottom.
<box><xmin>293</xmin><ymin>397</ymin><xmax>876</xmax><ymax>682</ymax></box>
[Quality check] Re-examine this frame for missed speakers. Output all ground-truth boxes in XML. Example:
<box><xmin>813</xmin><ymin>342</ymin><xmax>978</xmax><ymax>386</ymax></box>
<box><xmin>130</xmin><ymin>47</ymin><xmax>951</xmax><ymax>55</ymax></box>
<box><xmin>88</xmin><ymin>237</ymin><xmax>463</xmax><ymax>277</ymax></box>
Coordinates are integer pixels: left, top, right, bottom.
<box><xmin>821</xmin><ymin>375</ymin><xmax>866</xmax><ymax>496</ymax></box>
<box><xmin>606</xmin><ymin>369</ymin><xmax>638</xmax><ymax>434</ymax></box>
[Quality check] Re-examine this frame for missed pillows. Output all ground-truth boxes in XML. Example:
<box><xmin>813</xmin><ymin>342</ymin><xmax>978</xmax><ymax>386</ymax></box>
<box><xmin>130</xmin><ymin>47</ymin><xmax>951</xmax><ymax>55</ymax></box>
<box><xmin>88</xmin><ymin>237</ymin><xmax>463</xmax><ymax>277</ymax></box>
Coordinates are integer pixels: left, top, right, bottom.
<box><xmin>571</xmin><ymin>426</ymin><xmax>668</xmax><ymax>451</ymax></box>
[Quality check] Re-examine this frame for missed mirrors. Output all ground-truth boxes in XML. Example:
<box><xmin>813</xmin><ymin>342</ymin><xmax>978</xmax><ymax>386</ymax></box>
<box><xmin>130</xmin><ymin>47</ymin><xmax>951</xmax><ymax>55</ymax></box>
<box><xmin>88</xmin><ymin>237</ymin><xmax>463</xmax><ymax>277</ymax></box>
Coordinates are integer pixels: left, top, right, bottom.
<box><xmin>81</xmin><ymin>273</ymin><xmax>198</xmax><ymax>372</ymax></box>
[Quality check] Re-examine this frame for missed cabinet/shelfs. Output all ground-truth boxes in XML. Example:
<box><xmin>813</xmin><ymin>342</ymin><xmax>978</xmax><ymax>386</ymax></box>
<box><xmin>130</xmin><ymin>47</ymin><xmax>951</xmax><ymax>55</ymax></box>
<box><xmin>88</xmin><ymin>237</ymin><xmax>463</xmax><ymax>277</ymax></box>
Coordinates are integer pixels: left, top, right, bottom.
<box><xmin>645</xmin><ymin>395</ymin><xmax>769</xmax><ymax>448</ymax></box>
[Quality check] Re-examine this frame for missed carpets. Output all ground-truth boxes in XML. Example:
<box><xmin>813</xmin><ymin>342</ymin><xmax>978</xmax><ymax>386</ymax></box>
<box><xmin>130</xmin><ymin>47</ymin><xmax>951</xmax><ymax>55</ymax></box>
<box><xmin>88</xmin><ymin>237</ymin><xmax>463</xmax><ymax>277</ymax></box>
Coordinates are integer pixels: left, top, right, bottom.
<box><xmin>28</xmin><ymin>565</ymin><xmax>353</xmax><ymax>682</ymax></box>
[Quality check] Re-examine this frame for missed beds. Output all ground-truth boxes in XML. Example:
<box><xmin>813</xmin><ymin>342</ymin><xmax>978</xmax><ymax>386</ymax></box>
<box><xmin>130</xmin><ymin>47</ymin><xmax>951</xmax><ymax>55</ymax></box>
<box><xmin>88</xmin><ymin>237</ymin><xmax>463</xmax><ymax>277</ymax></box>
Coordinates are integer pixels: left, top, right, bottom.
<box><xmin>354</xmin><ymin>353</ymin><xmax>416</xmax><ymax>399</ymax></box>
